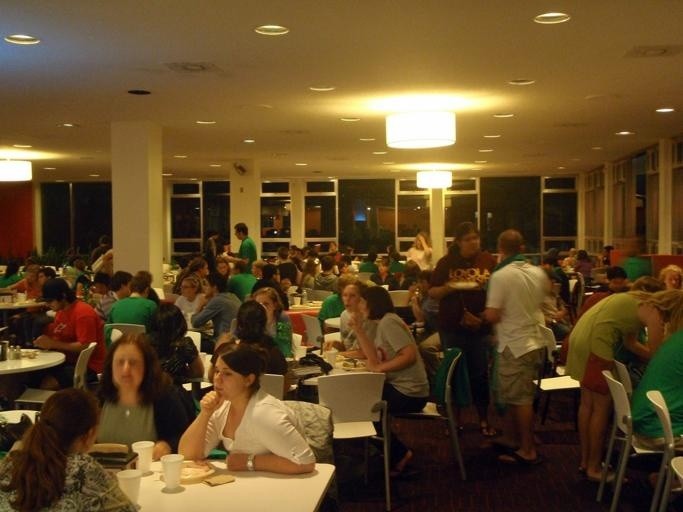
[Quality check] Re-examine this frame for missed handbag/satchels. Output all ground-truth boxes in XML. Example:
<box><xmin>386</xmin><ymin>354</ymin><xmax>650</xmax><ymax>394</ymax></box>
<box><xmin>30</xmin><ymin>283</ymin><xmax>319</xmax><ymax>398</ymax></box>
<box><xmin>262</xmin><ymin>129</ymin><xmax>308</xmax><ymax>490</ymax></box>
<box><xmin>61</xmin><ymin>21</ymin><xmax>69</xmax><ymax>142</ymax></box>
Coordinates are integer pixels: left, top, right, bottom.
<box><xmin>459</xmin><ymin>310</ymin><xmax>483</xmax><ymax>334</ymax></box>
<box><xmin>0</xmin><ymin>412</ymin><xmax>31</xmax><ymax>451</ymax></box>
<box><xmin>581</xmin><ymin>352</ymin><xmax>614</xmax><ymax>395</ymax></box>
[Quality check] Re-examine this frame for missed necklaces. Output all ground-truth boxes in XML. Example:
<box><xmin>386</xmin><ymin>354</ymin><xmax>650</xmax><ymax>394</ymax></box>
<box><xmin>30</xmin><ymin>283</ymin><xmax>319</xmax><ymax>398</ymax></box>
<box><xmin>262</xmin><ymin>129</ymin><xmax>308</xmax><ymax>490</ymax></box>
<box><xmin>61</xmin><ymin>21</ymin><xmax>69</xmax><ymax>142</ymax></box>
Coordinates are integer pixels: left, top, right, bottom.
<box><xmin>126</xmin><ymin>408</ymin><xmax>131</xmax><ymax>416</ymax></box>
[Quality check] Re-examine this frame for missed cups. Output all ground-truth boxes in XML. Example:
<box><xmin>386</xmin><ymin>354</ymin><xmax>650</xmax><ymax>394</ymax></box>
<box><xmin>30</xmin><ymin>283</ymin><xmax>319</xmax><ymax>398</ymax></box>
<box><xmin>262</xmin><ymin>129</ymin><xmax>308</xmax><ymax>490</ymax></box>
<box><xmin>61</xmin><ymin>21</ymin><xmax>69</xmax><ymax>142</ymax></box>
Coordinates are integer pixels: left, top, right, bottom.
<box><xmin>131</xmin><ymin>441</ymin><xmax>154</xmax><ymax>472</ymax></box>
<box><xmin>116</xmin><ymin>469</ymin><xmax>140</xmax><ymax>506</ymax></box>
<box><xmin>294</xmin><ymin>297</ymin><xmax>300</xmax><ymax>305</ymax></box>
<box><xmin>292</xmin><ymin>305</ymin><xmax>309</xmax><ymax>308</ymax></box>
<box><xmin>296</xmin><ymin>346</ymin><xmax>307</xmax><ymax>359</ymax></box>
<box><xmin>326</xmin><ymin>351</ymin><xmax>336</xmax><ymax>366</ymax></box>
<box><xmin>160</xmin><ymin>454</ymin><xmax>184</xmax><ymax>489</ymax></box>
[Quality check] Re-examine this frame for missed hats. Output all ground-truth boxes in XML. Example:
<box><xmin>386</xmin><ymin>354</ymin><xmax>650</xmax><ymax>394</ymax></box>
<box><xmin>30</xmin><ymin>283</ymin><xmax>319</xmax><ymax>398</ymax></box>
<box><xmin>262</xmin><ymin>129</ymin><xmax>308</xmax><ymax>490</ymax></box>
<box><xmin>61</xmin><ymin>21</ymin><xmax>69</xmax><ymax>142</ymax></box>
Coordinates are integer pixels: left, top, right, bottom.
<box><xmin>35</xmin><ymin>278</ymin><xmax>68</xmax><ymax>302</ymax></box>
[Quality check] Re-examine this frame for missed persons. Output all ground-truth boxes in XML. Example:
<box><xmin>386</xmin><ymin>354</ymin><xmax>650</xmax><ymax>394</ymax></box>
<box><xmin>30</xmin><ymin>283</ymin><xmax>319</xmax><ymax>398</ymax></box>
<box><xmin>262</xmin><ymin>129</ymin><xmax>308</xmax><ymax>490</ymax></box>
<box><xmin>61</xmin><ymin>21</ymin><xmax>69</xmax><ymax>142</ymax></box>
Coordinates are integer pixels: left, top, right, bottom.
<box><xmin>426</xmin><ymin>220</ymin><xmax>499</xmax><ymax>438</ymax></box>
<box><xmin>178</xmin><ymin>343</ymin><xmax>316</xmax><ymax>475</ymax></box>
<box><xmin>481</xmin><ymin>229</ymin><xmax>553</xmax><ymax>467</ymax></box>
<box><xmin>630</xmin><ymin>298</ymin><xmax>683</xmax><ymax>504</ymax></box>
<box><xmin>563</xmin><ymin>287</ymin><xmax>683</xmax><ymax>488</ymax></box>
<box><xmin>348</xmin><ymin>286</ymin><xmax>430</xmax><ymax>478</ymax></box>
<box><xmin>0</xmin><ymin>222</ymin><xmax>682</xmax><ymax>397</ymax></box>
<box><xmin>0</xmin><ymin>388</ymin><xmax>136</xmax><ymax>511</ymax></box>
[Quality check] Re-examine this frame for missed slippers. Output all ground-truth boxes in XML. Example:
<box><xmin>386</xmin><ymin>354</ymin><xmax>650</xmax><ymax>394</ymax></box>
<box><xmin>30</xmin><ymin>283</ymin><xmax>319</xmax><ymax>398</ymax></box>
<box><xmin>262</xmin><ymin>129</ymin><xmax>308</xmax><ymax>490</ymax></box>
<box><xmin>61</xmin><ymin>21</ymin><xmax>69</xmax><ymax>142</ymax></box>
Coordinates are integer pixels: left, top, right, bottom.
<box><xmin>575</xmin><ymin>462</ymin><xmax>612</xmax><ymax>473</ymax></box>
<box><xmin>497</xmin><ymin>450</ymin><xmax>536</xmax><ymax>465</ymax></box>
<box><xmin>585</xmin><ymin>472</ymin><xmax>628</xmax><ymax>486</ymax></box>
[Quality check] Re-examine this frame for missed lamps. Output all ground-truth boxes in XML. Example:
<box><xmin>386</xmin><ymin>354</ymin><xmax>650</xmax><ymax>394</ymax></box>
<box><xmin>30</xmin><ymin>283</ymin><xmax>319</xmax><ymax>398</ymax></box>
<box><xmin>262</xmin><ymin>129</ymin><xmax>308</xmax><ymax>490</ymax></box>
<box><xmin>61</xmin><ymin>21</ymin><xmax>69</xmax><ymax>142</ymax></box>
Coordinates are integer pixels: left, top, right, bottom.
<box><xmin>416</xmin><ymin>171</ymin><xmax>453</xmax><ymax>189</ymax></box>
<box><xmin>0</xmin><ymin>159</ymin><xmax>33</xmax><ymax>182</ymax></box>
<box><xmin>386</xmin><ymin>110</ymin><xmax>457</xmax><ymax>150</ymax></box>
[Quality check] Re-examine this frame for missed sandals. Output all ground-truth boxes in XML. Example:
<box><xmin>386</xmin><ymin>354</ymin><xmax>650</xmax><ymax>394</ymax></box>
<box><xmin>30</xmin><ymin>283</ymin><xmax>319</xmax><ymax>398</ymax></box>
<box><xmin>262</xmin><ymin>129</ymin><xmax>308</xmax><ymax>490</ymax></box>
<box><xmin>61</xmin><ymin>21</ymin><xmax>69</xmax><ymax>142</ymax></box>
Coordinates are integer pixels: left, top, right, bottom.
<box><xmin>478</xmin><ymin>425</ymin><xmax>487</xmax><ymax>436</ymax></box>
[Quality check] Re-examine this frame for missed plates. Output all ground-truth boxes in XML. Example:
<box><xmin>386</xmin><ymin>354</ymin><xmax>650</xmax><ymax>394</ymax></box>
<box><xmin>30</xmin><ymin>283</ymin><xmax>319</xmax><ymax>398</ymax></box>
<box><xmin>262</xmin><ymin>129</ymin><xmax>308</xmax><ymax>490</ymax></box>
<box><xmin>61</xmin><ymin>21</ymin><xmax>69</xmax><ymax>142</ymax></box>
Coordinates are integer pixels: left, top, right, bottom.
<box><xmin>177</xmin><ymin>461</ymin><xmax>215</xmax><ymax>479</ymax></box>
<box><xmin>343</xmin><ymin>366</ymin><xmax>366</xmax><ymax>370</ymax></box>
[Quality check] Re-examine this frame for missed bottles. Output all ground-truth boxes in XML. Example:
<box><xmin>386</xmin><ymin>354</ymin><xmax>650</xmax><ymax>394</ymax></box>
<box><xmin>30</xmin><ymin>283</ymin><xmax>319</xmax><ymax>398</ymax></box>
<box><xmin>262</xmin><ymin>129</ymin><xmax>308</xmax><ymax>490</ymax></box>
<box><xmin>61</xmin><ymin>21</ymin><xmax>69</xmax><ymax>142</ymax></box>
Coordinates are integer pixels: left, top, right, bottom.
<box><xmin>0</xmin><ymin>341</ymin><xmax>20</xmax><ymax>361</ymax></box>
<box><xmin>302</xmin><ymin>290</ymin><xmax>307</xmax><ymax>301</ymax></box>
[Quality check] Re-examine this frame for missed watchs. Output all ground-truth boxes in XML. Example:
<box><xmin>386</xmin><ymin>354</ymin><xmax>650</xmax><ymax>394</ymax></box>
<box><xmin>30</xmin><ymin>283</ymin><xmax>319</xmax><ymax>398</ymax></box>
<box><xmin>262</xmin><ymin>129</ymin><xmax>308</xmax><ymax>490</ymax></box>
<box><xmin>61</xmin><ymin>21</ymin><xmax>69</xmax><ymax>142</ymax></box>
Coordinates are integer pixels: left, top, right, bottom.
<box><xmin>246</xmin><ymin>454</ymin><xmax>256</xmax><ymax>473</ymax></box>
<box><xmin>92</xmin><ymin>332</ymin><xmax>189</xmax><ymax>461</ymax></box>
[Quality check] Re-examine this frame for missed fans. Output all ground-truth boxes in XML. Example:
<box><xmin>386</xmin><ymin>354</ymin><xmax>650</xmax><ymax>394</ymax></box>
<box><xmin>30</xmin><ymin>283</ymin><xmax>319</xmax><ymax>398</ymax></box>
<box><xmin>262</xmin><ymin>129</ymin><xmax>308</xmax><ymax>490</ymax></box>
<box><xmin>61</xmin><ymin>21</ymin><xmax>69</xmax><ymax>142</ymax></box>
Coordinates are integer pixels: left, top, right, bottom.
<box><xmin>233</xmin><ymin>162</ymin><xmax>245</xmax><ymax>176</ymax></box>
